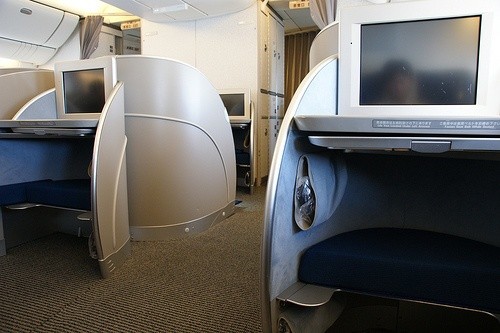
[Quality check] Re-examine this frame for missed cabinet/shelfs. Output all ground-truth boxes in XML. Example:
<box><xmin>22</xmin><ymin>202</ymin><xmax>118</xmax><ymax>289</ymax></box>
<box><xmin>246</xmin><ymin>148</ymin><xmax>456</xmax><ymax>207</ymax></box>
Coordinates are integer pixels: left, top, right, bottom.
<box><xmin>260</xmin><ymin>1</ymin><xmax>286</xmax><ymax>180</ymax></box>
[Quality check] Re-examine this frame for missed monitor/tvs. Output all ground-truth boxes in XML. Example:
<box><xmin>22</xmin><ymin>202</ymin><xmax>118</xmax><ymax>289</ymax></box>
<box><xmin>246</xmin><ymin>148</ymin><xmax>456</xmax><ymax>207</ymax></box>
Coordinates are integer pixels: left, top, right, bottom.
<box><xmin>54</xmin><ymin>56</ymin><xmax>117</xmax><ymax>119</ymax></box>
<box><xmin>339</xmin><ymin>1</ymin><xmax>500</xmax><ymax>118</ymax></box>
<box><xmin>216</xmin><ymin>88</ymin><xmax>251</xmax><ymax>123</ymax></box>
